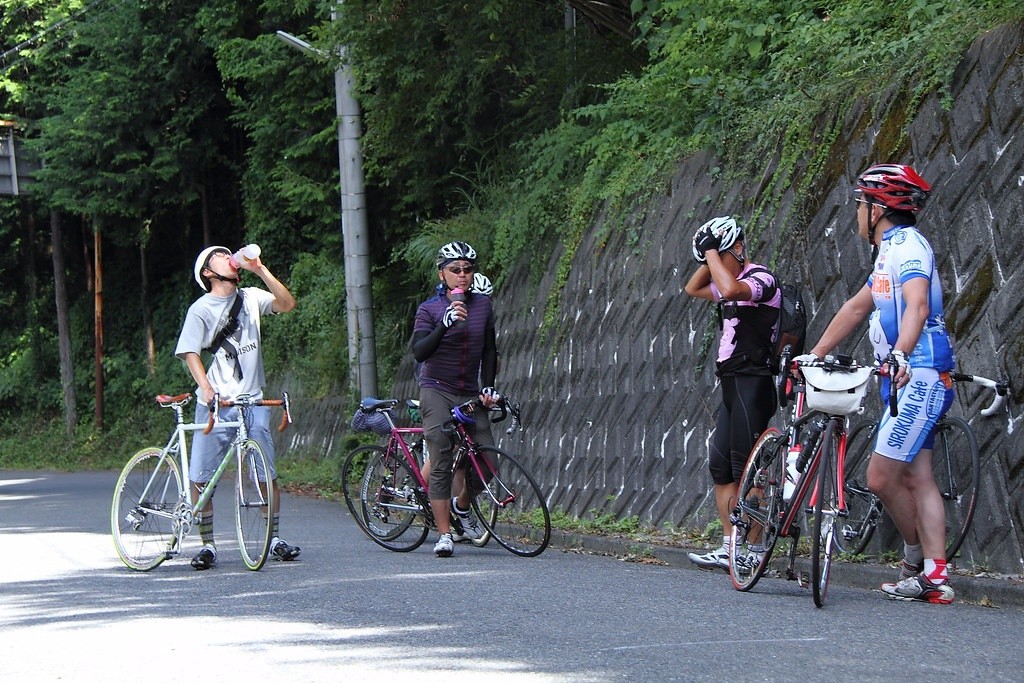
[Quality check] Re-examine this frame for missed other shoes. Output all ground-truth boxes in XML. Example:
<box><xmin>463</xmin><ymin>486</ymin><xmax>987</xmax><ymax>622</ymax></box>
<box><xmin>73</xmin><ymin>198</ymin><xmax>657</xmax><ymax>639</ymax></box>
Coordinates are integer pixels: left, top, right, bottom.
<box><xmin>450</xmin><ymin>527</ymin><xmax>470</xmax><ymax>541</ymax></box>
<box><xmin>269</xmin><ymin>541</ymin><xmax>301</xmax><ymax>560</ymax></box>
<box><xmin>191</xmin><ymin>548</ymin><xmax>218</xmax><ymax>567</ymax></box>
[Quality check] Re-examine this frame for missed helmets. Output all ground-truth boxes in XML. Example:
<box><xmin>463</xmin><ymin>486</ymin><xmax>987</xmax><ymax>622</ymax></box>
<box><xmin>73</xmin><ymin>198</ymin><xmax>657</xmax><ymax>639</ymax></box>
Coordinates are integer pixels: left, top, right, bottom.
<box><xmin>194</xmin><ymin>246</ymin><xmax>232</xmax><ymax>292</ymax></box>
<box><xmin>856</xmin><ymin>164</ymin><xmax>932</xmax><ymax>211</ymax></box>
<box><xmin>468</xmin><ymin>273</ymin><xmax>494</xmax><ymax>295</ymax></box>
<box><xmin>693</xmin><ymin>216</ymin><xmax>741</xmax><ymax>264</ymax></box>
<box><xmin>436</xmin><ymin>240</ymin><xmax>477</xmax><ymax>266</ymax></box>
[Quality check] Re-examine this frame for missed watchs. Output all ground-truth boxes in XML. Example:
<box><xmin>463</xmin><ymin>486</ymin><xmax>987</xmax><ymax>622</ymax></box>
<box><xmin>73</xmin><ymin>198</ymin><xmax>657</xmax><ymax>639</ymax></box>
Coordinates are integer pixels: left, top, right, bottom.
<box><xmin>892</xmin><ymin>350</ymin><xmax>909</xmax><ymax>362</ymax></box>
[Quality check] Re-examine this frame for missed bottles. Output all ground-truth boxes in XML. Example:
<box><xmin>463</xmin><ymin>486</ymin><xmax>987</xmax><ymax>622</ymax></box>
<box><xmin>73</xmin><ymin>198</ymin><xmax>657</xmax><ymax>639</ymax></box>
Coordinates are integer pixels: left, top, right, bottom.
<box><xmin>796</xmin><ymin>419</ymin><xmax>826</xmax><ymax>473</ymax></box>
<box><xmin>783</xmin><ymin>444</ymin><xmax>803</xmax><ymax>502</ymax></box>
<box><xmin>229</xmin><ymin>244</ymin><xmax>261</xmax><ymax>269</ymax></box>
<box><xmin>451</xmin><ymin>286</ymin><xmax>467</xmax><ymax>327</ymax></box>
<box><xmin>786</xmin><ymin>362</ymin><xmax>798</xmax><ymax>400</ymax></box>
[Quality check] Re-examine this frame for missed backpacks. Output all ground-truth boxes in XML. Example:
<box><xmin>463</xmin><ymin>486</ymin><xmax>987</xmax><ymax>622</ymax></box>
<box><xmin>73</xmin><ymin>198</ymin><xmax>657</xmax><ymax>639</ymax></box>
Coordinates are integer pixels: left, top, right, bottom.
<box><xmin>737</xmin><ymin>267</ymin><xmax>806</xmax><ymax>375</ymax></box>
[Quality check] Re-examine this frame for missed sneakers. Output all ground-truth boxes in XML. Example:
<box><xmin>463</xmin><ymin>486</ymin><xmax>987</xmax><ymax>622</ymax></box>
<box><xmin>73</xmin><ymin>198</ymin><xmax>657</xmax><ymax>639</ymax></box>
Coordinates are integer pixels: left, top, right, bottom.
<box><xmin>880</xmin><ymin>571</ymin><xmax>955</xmax><ymax>604</ymax></box>
<box><xmin>717</xmin><ymin>553</ymin><xmax>764</xmax><ymax>574</ymax></box>
<box><xmin>689</xmin><ymin>548</ymin><xmax>731</xmax><ymax>574</ymax></box>
<box><xmin>434</xmin><ymin>533</ymin><xmax>454</xmax><ymax>557</ymax></box>
<box><xmin>450</xmin><ymin>497</ymin><xmax>485</xmax><ymax>538</ymax></box>
<box><xmin>898</xmin><ymin>559</ymin><xmax>924</xmax><ymax>580</ymax></box>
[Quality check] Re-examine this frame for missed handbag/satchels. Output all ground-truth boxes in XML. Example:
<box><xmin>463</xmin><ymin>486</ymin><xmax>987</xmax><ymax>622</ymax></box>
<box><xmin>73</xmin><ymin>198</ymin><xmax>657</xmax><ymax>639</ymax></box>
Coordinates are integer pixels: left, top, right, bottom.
<box><xmin>351</xmin><ymin>409</ymin><xmax>397</xmax><ymax>434</ymax></box>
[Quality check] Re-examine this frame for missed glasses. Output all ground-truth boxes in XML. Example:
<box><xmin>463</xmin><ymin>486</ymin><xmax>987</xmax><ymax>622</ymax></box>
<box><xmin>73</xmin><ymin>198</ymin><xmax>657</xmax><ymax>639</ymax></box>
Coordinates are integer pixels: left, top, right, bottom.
<box><xmin>854</xmin><ymin>197</ymin><xmax>889</xmax><ymax>209</ymax></box>
<box><xmin>208</xmin><ymin>250</ymin><xmax>233</xmax><ymax>266</ymax></box>
<box><xmin>444</xmin><ymin>266</ymin><xmax>473</xmax><ymax>275</ymax></box>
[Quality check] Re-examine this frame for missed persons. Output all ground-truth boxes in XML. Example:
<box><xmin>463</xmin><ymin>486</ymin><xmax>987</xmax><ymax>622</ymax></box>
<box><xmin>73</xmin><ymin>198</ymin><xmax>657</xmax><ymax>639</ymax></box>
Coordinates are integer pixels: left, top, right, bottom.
<box><xmin>793</xmin><ymin>163</ymin><xmax>956</xmax><ymax>603</ymax></box>
<box><xmin>175</xmin><ymin>246</ymin><xmax>302</xmax><ymax>567</ymax></box>
<box><xmin>411</xmin><ymin>241</ymin><xmax>500</xmax><ymax>555</ymax></box>
<box><xmin>685</xmin><ymin>216</ymin><xmax>781</xmax><ymax>575</ymax></box>
